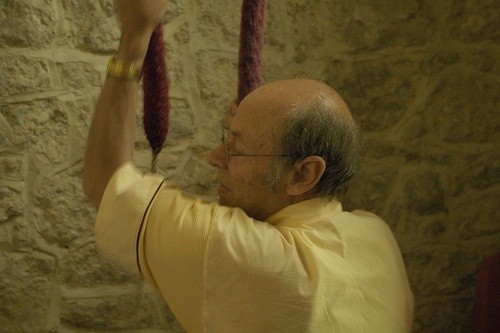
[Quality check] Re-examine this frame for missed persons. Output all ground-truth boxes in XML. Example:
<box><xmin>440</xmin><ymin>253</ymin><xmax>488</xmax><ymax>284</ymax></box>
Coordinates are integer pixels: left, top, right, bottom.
<box><xmin>81</xmin><ymin>0</ymin><xmax>416</xmax><ymax>333</ymax></box>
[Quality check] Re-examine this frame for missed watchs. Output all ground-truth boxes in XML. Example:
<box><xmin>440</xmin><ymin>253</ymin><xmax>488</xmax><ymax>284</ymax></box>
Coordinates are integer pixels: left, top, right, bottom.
<box><xmin>104</xmin><ymin>54</ymin><xmax>145</xmax><ymax>80</ymax></box>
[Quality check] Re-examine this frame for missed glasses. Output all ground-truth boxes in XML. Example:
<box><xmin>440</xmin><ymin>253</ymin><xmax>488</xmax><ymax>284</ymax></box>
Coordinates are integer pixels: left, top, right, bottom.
<box><xmin>221</xmin><ymin>127</ymin><xmax>309</xmax><ymax>170</ymax></box>
